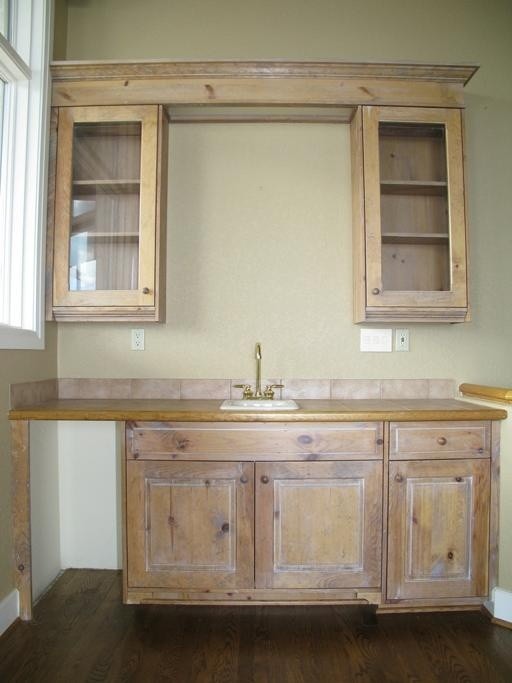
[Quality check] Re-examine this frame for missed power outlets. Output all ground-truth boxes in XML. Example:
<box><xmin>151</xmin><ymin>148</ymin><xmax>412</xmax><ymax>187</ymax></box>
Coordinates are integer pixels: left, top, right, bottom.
<box><xmin>130</xmin><ymin>327</ymin><xmax>146</xmax><ymax>351</ymax></box>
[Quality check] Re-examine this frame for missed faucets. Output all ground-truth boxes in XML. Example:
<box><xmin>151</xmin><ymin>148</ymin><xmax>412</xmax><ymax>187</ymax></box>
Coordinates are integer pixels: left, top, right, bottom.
<box><xmin>254</xmin><ymin>343</ymin><xmax>262</xmax><ymax>397</ymax></box>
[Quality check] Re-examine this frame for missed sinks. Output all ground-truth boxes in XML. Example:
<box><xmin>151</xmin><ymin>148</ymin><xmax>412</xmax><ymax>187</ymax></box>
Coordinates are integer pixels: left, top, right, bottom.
<box><xmin>219</xmin><ymin>400</ymin><xmax>300</xmax><ymax>412</ymax></box>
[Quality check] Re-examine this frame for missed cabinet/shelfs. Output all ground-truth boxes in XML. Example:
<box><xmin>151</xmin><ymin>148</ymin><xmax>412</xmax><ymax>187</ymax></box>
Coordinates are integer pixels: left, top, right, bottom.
<box><xmin>123</xmin><ymin>419</ymin><xmax>383</xmax><ymax>604</ymax></box>
<box><xmin>349</xmin><ymin>105</ymin><xmax>472</xmax><ymax>325</ymax></box>
<box><xmin>374</xmin><ymin>420</ymin><xmax>488</xmax><ymax>616</ymax></box>
<box><xmin>50</xmin><ymin>105</ymin><xmax>169</xmax><ymax>324</ymax></box>
